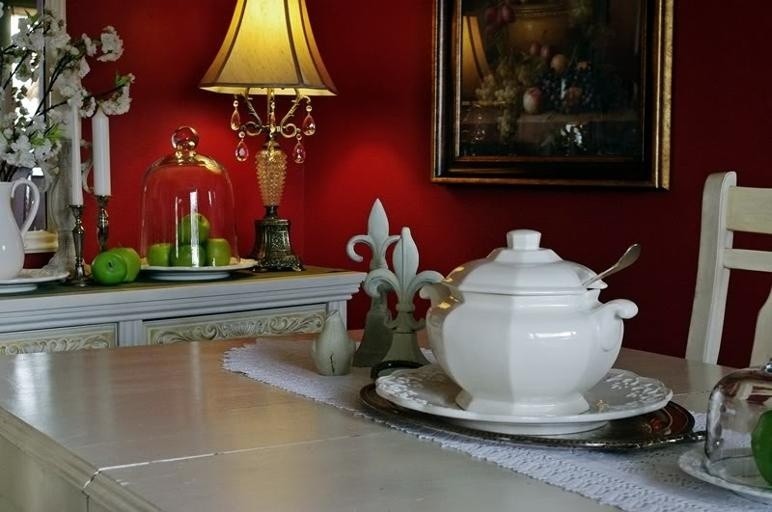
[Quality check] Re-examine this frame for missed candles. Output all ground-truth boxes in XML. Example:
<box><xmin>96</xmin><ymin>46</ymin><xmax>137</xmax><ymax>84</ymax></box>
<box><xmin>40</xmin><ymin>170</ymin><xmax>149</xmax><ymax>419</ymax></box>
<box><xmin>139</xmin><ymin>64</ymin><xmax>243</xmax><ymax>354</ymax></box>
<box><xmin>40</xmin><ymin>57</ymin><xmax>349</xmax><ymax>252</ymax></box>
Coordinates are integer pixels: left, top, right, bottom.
<box><xmin>91</xmin><ymin>105</ymin><xmax>113</xmax><ymax>196</ymax></box>
<box><xmin>69</xmin><ymin>103</ymin><xmax>83</xmax><ymax>207</ymax></box>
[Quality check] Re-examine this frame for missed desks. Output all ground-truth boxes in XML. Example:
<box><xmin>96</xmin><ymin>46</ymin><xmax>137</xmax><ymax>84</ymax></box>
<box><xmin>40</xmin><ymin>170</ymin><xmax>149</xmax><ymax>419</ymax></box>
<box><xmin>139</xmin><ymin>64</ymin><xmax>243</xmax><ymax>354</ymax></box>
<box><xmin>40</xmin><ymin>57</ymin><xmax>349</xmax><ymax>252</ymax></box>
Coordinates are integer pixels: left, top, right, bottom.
<box><xmin>83</xmin><ymin>390</ymin><xmax>771</xmax><ymax>511</ymax></box>
<box><xmin>0</xmin><ymin>329</ymin><xmax>739</xmax><ymax>511</ymax></box>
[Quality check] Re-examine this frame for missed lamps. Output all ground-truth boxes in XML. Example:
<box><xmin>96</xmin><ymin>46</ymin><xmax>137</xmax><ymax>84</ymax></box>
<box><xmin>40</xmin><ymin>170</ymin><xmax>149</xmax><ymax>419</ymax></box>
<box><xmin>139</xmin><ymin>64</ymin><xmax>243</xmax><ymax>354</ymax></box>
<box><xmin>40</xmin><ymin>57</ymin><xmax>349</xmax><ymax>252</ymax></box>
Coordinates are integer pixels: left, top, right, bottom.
<box><xmin>199</xmin><ymin>0</ymin><xmax>340</xmax><ymax>273</ymax></box>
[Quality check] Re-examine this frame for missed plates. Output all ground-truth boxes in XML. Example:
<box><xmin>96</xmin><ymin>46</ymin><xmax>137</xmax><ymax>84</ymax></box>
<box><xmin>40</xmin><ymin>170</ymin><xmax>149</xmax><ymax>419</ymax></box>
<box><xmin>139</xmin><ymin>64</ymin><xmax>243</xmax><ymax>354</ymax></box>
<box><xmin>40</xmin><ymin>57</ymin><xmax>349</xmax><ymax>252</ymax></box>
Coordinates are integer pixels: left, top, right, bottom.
<box><xmin>140</xmin><ymin>256</ymin><xmax>259</xmax><ymax>282</ymax></box>
<box><xmin>0</xmin><ymin>268</ymin><xmax>72</xmax><ymax>293</ymax></box>
<box><xmin>375</xmin><ymin>361</ymin><xmax>672</xmax><ymax>437</ymax></box>
<box><xmin>359</xmin><ymin>382</ymin><xmax>696</xmax><ymax>449</ymax></box>
<box><xmin>677</xmin><ymin>446</ymin><xmax>772</xmax><ymax>501</ymax></box>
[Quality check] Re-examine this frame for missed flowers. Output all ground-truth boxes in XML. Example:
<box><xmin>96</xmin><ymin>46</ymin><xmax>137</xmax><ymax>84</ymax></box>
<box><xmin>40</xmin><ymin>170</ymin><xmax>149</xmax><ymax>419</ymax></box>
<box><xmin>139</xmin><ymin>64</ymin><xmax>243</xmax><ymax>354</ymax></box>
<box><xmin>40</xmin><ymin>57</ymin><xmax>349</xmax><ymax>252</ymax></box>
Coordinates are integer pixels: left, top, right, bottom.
<box><xmin>0</xmin><ymin>0</ymin><xmax>136</xmax><ymax>182</ymax></box>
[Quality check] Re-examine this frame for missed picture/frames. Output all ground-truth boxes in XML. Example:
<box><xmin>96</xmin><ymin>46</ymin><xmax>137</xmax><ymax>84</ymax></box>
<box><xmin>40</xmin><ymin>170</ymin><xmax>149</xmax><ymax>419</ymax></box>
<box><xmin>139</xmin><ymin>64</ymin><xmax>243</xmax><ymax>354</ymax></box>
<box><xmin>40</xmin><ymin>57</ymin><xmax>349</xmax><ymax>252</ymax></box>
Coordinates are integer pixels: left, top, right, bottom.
<box><xmin>430</xmin><ymin>0</ymin><xmax>675</xmax><ymax>190</ymax></box>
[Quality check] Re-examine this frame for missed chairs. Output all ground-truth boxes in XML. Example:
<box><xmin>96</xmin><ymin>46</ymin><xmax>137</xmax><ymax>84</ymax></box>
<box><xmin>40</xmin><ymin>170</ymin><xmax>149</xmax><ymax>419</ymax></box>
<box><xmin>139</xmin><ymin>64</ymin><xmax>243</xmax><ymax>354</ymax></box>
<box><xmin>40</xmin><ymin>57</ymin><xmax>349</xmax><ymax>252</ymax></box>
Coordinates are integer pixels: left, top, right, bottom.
<box><xmin>686</xmin><ymin>172</ymin><xmax>772</xmax><ymax>368</ymax></box>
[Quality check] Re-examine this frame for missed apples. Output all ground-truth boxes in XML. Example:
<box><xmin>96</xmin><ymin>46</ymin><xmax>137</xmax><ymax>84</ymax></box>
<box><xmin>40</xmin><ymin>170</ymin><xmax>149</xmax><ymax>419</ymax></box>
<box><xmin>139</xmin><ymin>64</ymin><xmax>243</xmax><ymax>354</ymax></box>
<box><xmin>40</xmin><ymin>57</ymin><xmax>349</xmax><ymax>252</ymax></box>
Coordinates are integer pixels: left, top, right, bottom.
<box><xmin>178</xmin><ymin>213</ymin><xmax>211</xmax><ymax>245</ymax></box>
<box><xmin>91</xmin><ymin>251</ymin><xmax>127</xmax><ymax>284</ymax></box>
<box><xmin>107</xmin><ymin>246</ymin><xmax>141</xmax><ymax>283</ymax></box>
<box><xmin>749</xmin><ymin>411</ymin><xmax>772</xmax><ymax>485</ymax></box>
<box><xmin>147</xmin><ymin>242</ymin><xmax>174</xmax><ymax>267</ymax></box>
<box><xmin>205</xmin><ymin>238</ymin><xmax>231</xmax><ymax>266</ymax></box>
<box><xmin>170</xmin><ymin>244</ymin><xmax>206</xmax><ymax>267</ymax></box>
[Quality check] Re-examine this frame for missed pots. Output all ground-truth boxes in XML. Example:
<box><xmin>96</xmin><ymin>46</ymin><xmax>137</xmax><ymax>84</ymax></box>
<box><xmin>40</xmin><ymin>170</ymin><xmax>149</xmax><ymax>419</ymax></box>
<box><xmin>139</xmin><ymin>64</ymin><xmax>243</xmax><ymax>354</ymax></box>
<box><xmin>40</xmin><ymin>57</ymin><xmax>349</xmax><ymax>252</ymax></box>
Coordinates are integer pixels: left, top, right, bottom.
<box><xmin>419</xmin><ymin>229</ymin><xmax>638</xmax><ymax>417</ymax></box>
